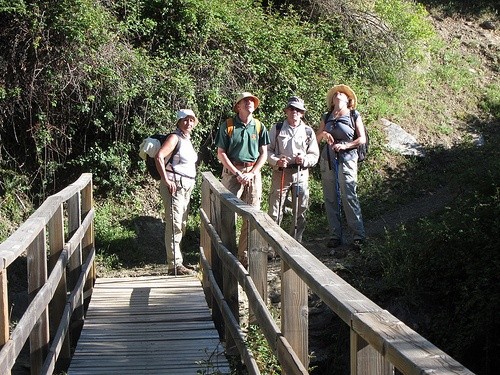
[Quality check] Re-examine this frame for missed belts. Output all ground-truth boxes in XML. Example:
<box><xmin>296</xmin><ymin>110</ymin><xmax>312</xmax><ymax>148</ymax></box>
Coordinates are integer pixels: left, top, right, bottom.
<box><xmin>287</xmin><ymin>164</ymin><xmax>298</xmax><ymax>168</ymax></box>
<box><xmin>232</xmin><ymin>160</ymin><xmax>255</xmax><ymax>167</ymax></box>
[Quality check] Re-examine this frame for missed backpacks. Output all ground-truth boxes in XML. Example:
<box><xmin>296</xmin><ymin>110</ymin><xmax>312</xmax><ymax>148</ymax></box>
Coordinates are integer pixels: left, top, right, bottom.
<box><xmin>324</xmin><ymin>110</ymin><xmax>370</xmax><ymax>169</ymax></box>
<box><xmin>145</xmin><ymin>133</ymin><xmax>181</xmax><ymax>181</ymax></box>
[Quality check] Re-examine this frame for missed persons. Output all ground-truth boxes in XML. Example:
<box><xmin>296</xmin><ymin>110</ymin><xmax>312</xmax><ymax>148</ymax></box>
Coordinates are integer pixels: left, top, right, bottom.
<box><xmin>268</xmin><ymin>97</ymin><xmax>319</xmax><ymax>260</ymax></box>
<box><xmin>219</xmin><ymin>92</ymin><xmax>271</xmax><ymax>266</ymax></box>
<box><xmin>156</xmin><ymin>109</ymin><xmax>198</xmax><ymax>274</ymax></box>
<box><xmin>316</xmin><ymin>85</ymin><xmax>365</xmax><ymax>247</ymax></box>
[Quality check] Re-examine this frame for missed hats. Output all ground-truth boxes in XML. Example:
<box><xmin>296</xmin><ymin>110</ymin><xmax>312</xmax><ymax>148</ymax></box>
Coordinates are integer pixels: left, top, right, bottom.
<box><xmin>174</xmin><ymin>109</ymin><xmax>198</xmax><ymax>127</ymax></box>
<box><xmin>325</xmin><ymin>84</ymin><xmax>357</xmax><ymax>110</ymax></box>
<box><xmin>232</xmin><ymin>91</ymin><xmax>260</xmax><ymax>113</ymax></box>
<box><xmin>285</xmin><ymin>96</ymin><xmax>306</xmax><ymax>111</ymax></box>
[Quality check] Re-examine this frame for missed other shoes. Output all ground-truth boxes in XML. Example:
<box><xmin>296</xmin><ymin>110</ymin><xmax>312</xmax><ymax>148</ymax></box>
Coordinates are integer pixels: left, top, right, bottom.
<box><xmin>354</xmin><ymin>239</ymin><xmax>362</xmax><ymax>244</ymax></box>
<box><xmin>327</xmin><ymin>239</ymin><xmax>341</xmax><ymax>248</ymax></box>
<box><xmin>168</xmin><ymin>266</ymin><xmax>192</xmax><ymax>275</ymax></box>
